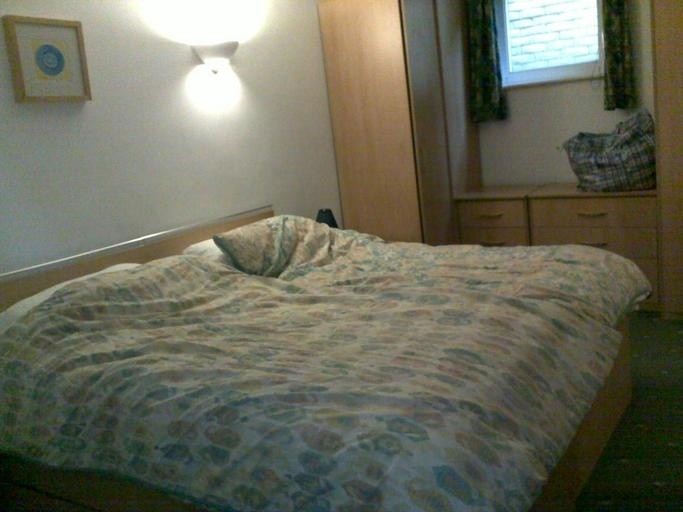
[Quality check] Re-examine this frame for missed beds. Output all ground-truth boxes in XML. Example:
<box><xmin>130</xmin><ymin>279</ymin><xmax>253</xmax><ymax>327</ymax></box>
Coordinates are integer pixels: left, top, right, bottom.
<box><xmin>0</xmin><ymin>206</ymin><xmax>633</xmax><ymax>512</ymax></box>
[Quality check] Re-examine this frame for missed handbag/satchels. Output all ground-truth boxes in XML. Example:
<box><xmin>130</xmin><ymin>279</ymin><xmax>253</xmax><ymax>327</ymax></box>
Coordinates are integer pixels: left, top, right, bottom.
<box><xmin>561</xmin><ymin>110</ymin><xmax>656</xmax><ymax>192</ymax></box>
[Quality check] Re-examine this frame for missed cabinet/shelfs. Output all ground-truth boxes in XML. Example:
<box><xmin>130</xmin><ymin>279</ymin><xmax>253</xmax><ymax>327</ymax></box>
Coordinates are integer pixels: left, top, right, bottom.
<box><xmin>318</xmin><ymin>0</ymin><xmax>481</xmax><ymax>245</ymax></box>
<box><xmin>632</xmin><ymin>0</ymin><xmax>683</xmax><ymax>321</ymax></box>
<box><xmin>455</xmin><ymin>181</ymin><xmax>657</xmax><ymax>318</ymax></box>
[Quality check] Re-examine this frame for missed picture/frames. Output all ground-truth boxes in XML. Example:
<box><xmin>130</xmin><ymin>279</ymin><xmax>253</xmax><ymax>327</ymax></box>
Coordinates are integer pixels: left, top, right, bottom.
<box><xmin>0</xmin><ymin>14</ymin><xmax>93</xmax><ymax>104</ymax></box>
